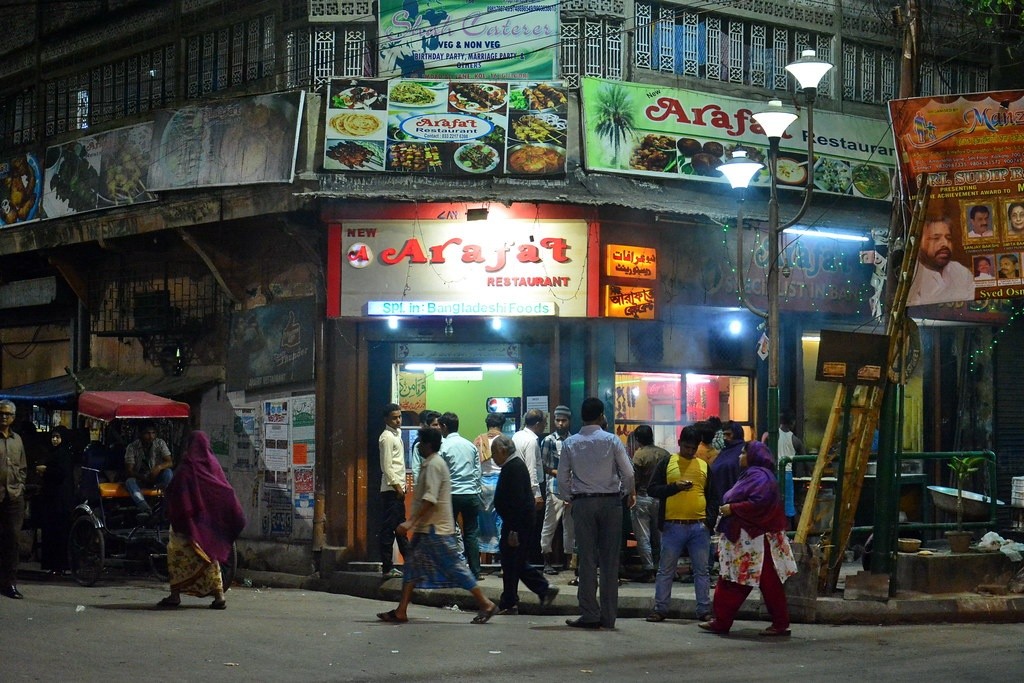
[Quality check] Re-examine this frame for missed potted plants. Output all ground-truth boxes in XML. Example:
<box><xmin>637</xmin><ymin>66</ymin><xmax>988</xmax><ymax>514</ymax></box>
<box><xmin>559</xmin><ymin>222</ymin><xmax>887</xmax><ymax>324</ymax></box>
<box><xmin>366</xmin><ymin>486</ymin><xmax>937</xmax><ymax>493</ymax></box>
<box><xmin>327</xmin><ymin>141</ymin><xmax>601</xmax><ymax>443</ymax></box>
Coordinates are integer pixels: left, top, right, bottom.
<box><xmin>943</xmin><ymin>456</ymin><xmax>996</xmax><ymax>552</ymax></box>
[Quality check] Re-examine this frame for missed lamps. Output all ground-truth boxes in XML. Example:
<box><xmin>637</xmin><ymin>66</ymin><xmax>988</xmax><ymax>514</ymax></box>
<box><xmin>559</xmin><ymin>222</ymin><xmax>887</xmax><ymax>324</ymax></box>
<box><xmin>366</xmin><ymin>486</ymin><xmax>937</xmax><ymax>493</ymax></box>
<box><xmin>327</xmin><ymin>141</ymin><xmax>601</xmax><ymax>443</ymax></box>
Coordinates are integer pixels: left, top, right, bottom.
<box><xmin>444</xmin><ymin>317</ymin><xmax>455</xmax><ymax>338</ymax></box>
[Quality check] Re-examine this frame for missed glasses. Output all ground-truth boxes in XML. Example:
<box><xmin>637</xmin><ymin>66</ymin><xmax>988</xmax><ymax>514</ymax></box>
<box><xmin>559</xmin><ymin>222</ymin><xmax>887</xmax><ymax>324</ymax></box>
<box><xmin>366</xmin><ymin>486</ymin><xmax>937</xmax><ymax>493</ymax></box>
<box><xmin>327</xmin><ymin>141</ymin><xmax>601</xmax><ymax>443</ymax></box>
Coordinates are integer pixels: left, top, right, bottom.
<box><xmin>0</xmin><ymin>412</ymin><xmax>15</xmax><ymax>417</ymax></box>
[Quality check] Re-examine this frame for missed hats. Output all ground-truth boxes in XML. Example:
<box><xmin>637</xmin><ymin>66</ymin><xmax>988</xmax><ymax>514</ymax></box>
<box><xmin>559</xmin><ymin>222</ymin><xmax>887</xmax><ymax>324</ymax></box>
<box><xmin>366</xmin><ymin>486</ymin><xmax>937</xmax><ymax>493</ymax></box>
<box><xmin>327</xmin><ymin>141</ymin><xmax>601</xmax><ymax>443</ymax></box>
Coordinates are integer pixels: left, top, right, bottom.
<box><xmin>553</xmin><ymin>405</ymin><xmax>572</xmax><ymax>417</ymax></box>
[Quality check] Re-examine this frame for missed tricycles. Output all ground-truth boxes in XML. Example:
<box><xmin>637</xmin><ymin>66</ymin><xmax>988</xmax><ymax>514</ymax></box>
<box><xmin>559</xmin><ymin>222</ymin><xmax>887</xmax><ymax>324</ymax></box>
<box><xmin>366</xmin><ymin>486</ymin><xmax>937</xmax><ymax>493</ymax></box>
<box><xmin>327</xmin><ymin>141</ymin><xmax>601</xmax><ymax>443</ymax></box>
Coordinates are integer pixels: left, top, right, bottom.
<box><xmin>66</xmin><ymin>390</ymin><xmax>238</xmax><ymax>594</ymax></box>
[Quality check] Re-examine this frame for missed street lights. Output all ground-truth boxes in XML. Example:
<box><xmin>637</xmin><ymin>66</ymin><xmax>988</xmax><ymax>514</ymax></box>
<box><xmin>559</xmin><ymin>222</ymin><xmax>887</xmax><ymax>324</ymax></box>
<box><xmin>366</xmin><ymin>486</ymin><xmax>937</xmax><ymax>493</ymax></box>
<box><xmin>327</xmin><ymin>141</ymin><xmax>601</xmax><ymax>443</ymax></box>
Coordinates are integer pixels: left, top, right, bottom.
<box><xmin>715</xmin><ymin>48</ymin><xmax>833</xmax><ymax>473</ymax></box>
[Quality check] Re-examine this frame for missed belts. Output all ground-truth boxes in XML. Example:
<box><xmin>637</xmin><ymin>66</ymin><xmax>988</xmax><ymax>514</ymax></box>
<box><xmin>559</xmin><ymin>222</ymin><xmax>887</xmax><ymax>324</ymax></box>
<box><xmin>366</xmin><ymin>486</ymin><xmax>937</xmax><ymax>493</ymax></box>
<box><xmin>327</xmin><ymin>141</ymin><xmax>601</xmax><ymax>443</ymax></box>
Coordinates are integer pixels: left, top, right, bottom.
<box><xmin>665</xmin><ymin>518</ymin><xmax>706</xmax><ymax>525</ymax></box>
<box><xmin>573</xmin><ymin>493</ymin><xmax>620</xmax><ymax>498</ymax></box>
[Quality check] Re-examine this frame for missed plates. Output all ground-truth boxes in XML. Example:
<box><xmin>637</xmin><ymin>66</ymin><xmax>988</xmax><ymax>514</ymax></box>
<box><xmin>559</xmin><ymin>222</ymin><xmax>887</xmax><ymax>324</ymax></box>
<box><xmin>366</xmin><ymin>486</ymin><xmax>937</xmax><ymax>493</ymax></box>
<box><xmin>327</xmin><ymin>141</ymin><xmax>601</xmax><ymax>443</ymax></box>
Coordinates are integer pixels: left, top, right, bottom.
<box><xmin>813</xmin><ymin>158</ymin><xmax>851</xmax><ymax>194</ymax></box>
<box><xmin>387</xmin><ymin>112</ymin><xmax>424</xmax><ymax>142</ymax></box>
<box><xmin>511</xmin><ymin>86</ymin><xmax>561</xmax><ymax>113</ymax></box>
<box><xmin>339</xmin><ymin>87</ymin><xmax>374</xmax><ymax>108</ymax></box>
<box><xmin>450</xmin><ymin>82</ymin><xmax>507</xmax><ymax>113</ymax></box>
<box><xmin>452</xmin><ymin>142</ymin><xmax>498</xmax><ymax>173</ymax></box>
<box><xmin>328</xmin><ymin>109</ymin><xmax>387</xmax><ymax>141</ymax></box>
<box><xmin>508</xmin><ymin>122</ymin><xmax>564</xmax><ymax>142</ymax></box>
<box><xmin>388</xmin><ymin>84</ymin><xmax>445</xmax><ymax>107</ymax></box>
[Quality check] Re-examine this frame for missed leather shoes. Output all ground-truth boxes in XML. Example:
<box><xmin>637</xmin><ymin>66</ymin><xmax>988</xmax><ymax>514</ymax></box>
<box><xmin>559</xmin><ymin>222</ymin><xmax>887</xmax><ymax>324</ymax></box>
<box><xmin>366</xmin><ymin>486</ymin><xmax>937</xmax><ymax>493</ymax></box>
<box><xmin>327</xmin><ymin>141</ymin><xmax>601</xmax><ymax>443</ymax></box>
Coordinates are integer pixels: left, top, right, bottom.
<box><xmin>565</xmin><ymin>617</ymin><xmax>614</xmax><ymax>629</ymax></box>
<box><xmin>1</xmin><ymin>585</ymin><xmax>23</xmax><ymax>599</ymax></box>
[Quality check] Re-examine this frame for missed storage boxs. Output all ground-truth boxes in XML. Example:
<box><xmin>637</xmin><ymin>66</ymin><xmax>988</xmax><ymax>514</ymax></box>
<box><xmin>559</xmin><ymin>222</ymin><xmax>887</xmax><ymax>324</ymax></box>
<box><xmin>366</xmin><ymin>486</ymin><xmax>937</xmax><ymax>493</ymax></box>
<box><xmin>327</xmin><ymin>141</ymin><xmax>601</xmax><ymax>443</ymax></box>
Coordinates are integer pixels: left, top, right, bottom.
<box><xmin>1010</xmin><ymin>476</ymin><xmax>1024</xmax><ymax>508</ymax></box>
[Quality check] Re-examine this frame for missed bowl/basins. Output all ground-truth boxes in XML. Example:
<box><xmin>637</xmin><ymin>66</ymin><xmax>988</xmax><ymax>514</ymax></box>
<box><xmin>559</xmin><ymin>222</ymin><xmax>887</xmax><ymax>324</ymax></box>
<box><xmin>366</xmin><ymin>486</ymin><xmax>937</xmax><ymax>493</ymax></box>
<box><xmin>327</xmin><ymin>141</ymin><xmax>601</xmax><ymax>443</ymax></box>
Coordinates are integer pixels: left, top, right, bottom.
<box><xmin>507</xmin><ymin>142</ymin><xmax>565</xmax><ymax>174</ymax></box>
<box><xmin>852</xmin><ymin>164</ymin><xmax>892</xmax><ymax>200</ymax></box>
<box><xmin>776</xmin><ymin>157</ymin><xmax>807</xmax><ymax>185</ymax></box>
<box><xmin>900</xmin><ymin>538</ymin><xmax>921</xmax><ymax>553</ymax></box>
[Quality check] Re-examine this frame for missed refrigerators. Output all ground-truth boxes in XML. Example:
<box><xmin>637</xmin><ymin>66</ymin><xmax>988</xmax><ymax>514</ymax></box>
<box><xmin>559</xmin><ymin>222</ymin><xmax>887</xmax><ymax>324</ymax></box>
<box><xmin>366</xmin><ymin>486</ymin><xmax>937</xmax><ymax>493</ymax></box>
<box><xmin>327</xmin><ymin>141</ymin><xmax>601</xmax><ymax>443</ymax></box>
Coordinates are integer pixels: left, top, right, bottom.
<box><xmin>485</xmin><ymin>394</ymin><xmax>519</xmax><ymax>442</ymax></box>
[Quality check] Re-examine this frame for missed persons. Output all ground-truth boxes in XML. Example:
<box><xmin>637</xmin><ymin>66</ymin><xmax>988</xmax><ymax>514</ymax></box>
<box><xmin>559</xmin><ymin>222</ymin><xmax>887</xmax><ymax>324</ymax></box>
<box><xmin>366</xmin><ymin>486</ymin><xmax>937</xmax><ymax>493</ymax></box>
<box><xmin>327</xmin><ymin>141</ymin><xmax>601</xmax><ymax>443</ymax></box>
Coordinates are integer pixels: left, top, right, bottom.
<box><xmin>968</xmin><ymin>202</ymin><xmax>1024</xmax><ymax>280</ymax></box>
<box><xmin>378</xmin><ymin>428</ymin><xmax>502</xmax><ymax>623</ymax></box>
<box><xmin>156</xmin><ymin>431</ymin><xmax>246</xmax><ymax>610</ymax></box>
<box><xmin>377</xmin><ymin>404</ymin><xmax>812</xmax><ymax>578</ymax></box>
<box><xmin>491</xmin><ymin>434</ymin><xmax>558</xmax><ymax>614</ymax></box>
<box><xmin>1</xmin><ymin>399</ymin><xmax>28</xmax><ymax>598</ymax></box>
<box><xmin>125</xmin><ymin>422</ymin><xmax>173</xmax><ymax>520</ymax></box>
<box><xmin>904</xmin><ymin>212</ymin><xmax>976</xmax><ymax>304</ymax></box>
<box><xmin>696</xmin><ymin>438</ymin><xmax>798</xmax><ymax>637</ymax></box>
<box><xmin>558</xmin><ymin>398</ymin><xmax>636</xmax><ymax>629</ymax></box>
<box><xmin>36</xmin><ymin>424</ymin><xmax>75</xmax><ymax>580</ymax></box>
<box><xmin>645</xmin><ymin>424</ymin><xmax>719</xmax><ymax>621</ymax></box>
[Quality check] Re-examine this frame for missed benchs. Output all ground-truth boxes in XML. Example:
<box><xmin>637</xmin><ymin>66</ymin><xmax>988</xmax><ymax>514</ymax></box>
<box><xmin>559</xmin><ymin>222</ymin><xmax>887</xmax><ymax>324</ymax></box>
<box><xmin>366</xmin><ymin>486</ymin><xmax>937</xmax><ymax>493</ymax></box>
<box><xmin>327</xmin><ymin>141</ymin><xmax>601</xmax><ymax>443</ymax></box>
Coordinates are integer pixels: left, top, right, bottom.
<box><xmin>82</xmin><ymin>460</ymin><xmax>176</xmax><ymax>505</ymax></box>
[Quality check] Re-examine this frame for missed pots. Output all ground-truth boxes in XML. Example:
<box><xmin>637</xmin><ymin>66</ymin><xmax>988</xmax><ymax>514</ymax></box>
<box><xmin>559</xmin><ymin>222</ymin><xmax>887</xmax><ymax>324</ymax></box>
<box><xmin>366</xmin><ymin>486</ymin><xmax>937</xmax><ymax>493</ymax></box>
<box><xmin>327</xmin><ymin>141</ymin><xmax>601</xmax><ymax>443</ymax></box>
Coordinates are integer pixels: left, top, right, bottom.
<box><xmin>866</xmin><ymin>462</ymin><xmax>877</xmax><ymax>474</ymax></box>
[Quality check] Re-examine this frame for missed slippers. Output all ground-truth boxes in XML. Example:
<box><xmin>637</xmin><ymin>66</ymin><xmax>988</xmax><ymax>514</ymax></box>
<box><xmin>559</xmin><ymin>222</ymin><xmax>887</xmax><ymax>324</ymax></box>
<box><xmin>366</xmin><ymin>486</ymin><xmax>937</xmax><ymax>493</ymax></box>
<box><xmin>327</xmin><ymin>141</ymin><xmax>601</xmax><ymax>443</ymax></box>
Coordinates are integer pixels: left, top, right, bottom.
<box><xmin>469</xmin><ymin>605</ymin><xmax>500</xmax><ymax>624</ymax></box>
<box><xmin>698</xmin><ymin>621</ymin><xmax>729</xmax><ymax>634</ymax></box>
<box><xmin>383</xmin><ymin>568</ymin><xmax>403</xmax><ymax>580</ymax></box>
<box><xmin>209</xmin><ymin>600</ymin><xmax>227</xmax><ymax>610</ymax></box>
<box><xmin>377</xmin><ymin>609</ymin><xmax>409</xmax><ymax>623</ymax></box>
<box><xmin>157</xmin><ymin>598</ymin><xmax>181</xmax><ymax>609</ymax></box>
<box><xmin>759</xmin><ymin>626</ymin><xmax>791</xmax><ymax>636</ymax></box>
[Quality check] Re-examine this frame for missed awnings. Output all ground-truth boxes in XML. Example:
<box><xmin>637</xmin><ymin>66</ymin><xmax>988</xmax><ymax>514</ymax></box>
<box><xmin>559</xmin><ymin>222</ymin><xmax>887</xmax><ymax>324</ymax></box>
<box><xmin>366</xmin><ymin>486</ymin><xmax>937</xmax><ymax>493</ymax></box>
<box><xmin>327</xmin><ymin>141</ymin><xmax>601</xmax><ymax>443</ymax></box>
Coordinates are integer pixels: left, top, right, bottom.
<box><xmin>294</xmin><ymin>173</ymin><xmax>890</xmax><ymax>233</ymax></box>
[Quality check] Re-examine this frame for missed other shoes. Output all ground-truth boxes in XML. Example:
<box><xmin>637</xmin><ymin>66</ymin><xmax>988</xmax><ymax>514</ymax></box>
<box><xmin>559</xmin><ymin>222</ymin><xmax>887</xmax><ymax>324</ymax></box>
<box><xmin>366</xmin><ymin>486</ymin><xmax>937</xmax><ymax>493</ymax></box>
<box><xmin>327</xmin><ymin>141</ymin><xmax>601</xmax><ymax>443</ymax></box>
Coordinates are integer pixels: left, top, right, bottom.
<box><xmin>491</xmin><ymin>604</ymin><xmax>519</xmax><ymax>615</ymax></box>
<box><xmin>568</xmin><ymin>576</ymin><xmax>579</xmax><ymax>586</ymax></box>
<box><xmin>60</xmin><ymin>568</ymin><xmax>73</xmax><ymax>581</ymax></box>
<box><xmin>646</xmin><ymin>611</ymin><xmax>666</xmax><ymax>622</ymax></box>
<box><xmin>699</xmin><ymin>614</ymin><xmax>714</xmax><ymax>622</ymax></box>
<box><xmin>636</xmin><ymin>572</ymin><xmax>656</xmax><ymax>582</ymax></box>
<box><xmin>541</xmin><ymin>585</ymin><xmax>559</xmax><ymax>609</ymax></box>
<box><xmin>544</xmin><ymin>568</ymin><xmax>558</xmax><ymax>575</ymax></box>
